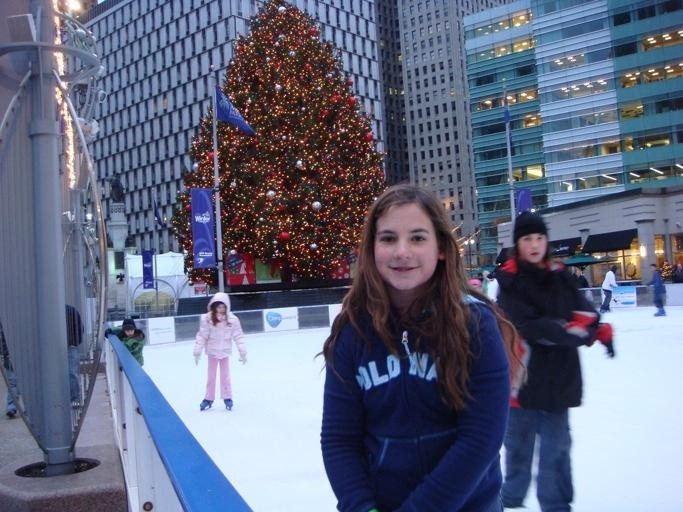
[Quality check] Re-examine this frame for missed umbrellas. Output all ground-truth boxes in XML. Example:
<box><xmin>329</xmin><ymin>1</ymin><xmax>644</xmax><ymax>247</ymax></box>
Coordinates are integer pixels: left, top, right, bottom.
<box><xmin>598</xmin><ymin>256</ymin><xmax>617</xmax><ymax>262</ymax></box>
<box><xmin>561</xmin><ymin>256</ymin><xmax>604</xmax><ymax>277</ymax></box>
<box><xmin>468</xmin><ymin>262</ymin><xmax>497</xmax><ymax>275</ymax></box>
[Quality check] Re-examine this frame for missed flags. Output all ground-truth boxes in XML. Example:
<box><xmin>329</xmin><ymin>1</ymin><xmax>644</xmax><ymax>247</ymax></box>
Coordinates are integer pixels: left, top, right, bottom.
<box><xmin>152</xmin><ymin>199</ymin><xmax>164</xmax><ymax>226</ymax></box>
<box><xmin>502</xmin><ymin>91</ymin><xmax>516</xmax><ymax>156</ymax></box>
<box><xmin>217</xmin><ymin>86</ymin><xmax>260</xmax><ymax>138</ymax></box>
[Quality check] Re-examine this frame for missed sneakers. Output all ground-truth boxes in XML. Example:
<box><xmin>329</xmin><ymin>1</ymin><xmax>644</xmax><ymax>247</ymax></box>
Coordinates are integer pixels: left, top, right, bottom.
<box><xmin>224</xmin><ymin>398</ymin><xmax>233</xmax><ymax>407</ymax></box>
<box><xmin>599</xmin><ymin>307</ymin><xmax>612</xmax><ymax>313</ymax></box>
<box><xmin>654</xmin><ymin>307</ymin><xmax>666</xmax><ymax>315</ymax></box>
<box><xmin>199</xmin><ymin>399</ymin><xmax>213</xmax><ymax>408</ymax></box>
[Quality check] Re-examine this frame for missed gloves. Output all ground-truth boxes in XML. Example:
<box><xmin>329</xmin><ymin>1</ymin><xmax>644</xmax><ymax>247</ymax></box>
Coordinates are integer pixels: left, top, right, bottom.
<box><xmin>194</xmin><ymin>354</ymin><xmax>200</xmax><ymax>366</ymax></box>
<box><xmin>563</xmin><ymin>311</ymin><xmax>615</xmax><ymax>358</ymax></box>
<box><xmin>105</xmin><ymin>328</ymin><xmax>112</xmax><ymax>339</ymax></box>
<box><xmin>239</xmin><ymin>354</ymin><xmax>248</xmax><ymax>364</ymax></box>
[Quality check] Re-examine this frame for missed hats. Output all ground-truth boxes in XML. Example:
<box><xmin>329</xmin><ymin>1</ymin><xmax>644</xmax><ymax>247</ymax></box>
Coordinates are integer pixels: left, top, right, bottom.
<box><xmin>512</xmin><ymin>211</ymin><xmax>548</xmax><ymax>243</ymax></box>
<box><xmin>122</xmin><ymin>319</ymin><xmax>136</xmax><ymax>329</ymax></box>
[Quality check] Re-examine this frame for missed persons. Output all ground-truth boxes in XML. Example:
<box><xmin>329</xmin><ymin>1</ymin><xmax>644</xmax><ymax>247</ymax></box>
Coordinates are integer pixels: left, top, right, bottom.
<box><xmin>671</xmin><ymin>263</ymin><xmax>683</xmax><ymax>284</ymax></box>
<box><xmin>644</xmin><ymin>263</ymin><xmax>666</xmax><ymax>317</ymax></box>
<box><xmin>485</xmin><ymin>210</ymin><xmax>614</xmax><ymax>512</ymax></box>
<box><xmin>105</xmin><ymin>318</ymin><xmax>146</xmax><ymax>367</ymax></box>
<box><xmin>598</xmin><ymin>264</ymin><xmax>618</xmax><ymax>312</ymax></box>
<box><xmin>66</xmin><ymin>341</ymin><xmax>82</xmax><ymax>408</ymax></box>
<box><xmin>190</xmin><ymin>291</ymin><xmax>246</xmax><ymax>409</ymax></box>
<box><xmin>1</xmin><ymin>363</ymin><xmax>18</xmax><ymax>417</ymax></box>
<box><xmin>312</xmin><ymin>182</ymin><xmax>529</xmax><ymax>512</ymax></box>
<box><xmin>467</xmin><ymin>279</ymin><xmax>482</xmax><ymax>295</ymax></box>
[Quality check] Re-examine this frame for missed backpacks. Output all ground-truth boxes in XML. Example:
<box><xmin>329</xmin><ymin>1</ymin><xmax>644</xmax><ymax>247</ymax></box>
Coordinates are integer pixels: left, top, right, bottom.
<box><xmin>486</xmin><ymin>296</ymin><xmax>530</xmax><ymax>408</ymax></box>
